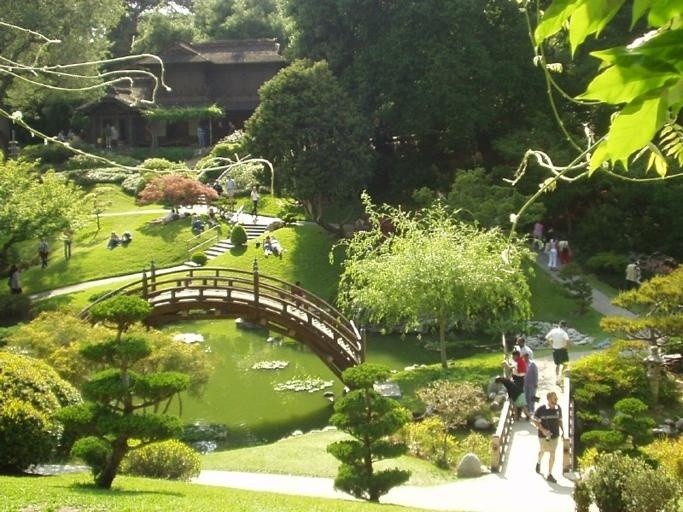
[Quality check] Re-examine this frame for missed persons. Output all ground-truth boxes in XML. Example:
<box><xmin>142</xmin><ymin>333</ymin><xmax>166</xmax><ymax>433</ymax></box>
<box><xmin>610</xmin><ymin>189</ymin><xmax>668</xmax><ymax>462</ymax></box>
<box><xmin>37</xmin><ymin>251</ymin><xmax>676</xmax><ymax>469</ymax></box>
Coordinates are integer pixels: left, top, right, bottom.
<box><xmin>625</xmin><ymin>261</ymin><xmax>640</xmax><ymax>292</ymax></box>
<box><xmin>56</xmin><ymin>122</ymin><xmax>119</xmax><ymax>148</ymax></box>
<box><xmin>251</xmin><ymin>186</ymin><xmax>259</xmax><ymax>217</ymax></box>
<box><xmin>107</xmin><ymin>230</ymin><xmax>132</xmax><ymax>249</ymax></box>
<box><xmin>39</xmin><ymin>239</ymin><xmax>49</xmax><ymax>268</ymax></box>
<box><xmin>543</xmin><ymin>319</ymin><xmax>569</xmax><ymax>388</ymax></box>
<box><xmin>534</xmin><ymin>211</ymin><xmax>576</xmax><ymax>270</ymax></box>
<box><xmin>292</xmin><ymin>281</ymin><xmax>305</xmax><ymax>315</ymax></box>
<box><xmin>533</xmin><ymin>392</ymin><xmax>566</xmax><ymax>483</ymax></box>
<box><xmin>264</xmin><ymin>234</ymin><xmax>283</xmax><ymax>257</ymax></box>
<box><xmin>196</xmin><ymin>119</ymin><xmax>235</xmax><ymax>147</ymax></box>
<box><xmin>64</xmin><ymin>225</ymin><xmax>73</xmax><ymax>259</ymax></box>
<box><xmin>355</xmin><ymin>216</ymin><xmax>365</xmax><ymax>227</ymax></box>
<box><xmin>9</xmin><ymin>265</ymin><xmax>21</xmax><ymax>295</ymax></box>
<box><xmin>148</xmin><ymin>175</ymin><xmax>237</xmax><ymax>232</ymax></box>
<box><xmin>494</xmin><ymin>336</ymin><xmax>540</xmax><ymax>422</ymax></box>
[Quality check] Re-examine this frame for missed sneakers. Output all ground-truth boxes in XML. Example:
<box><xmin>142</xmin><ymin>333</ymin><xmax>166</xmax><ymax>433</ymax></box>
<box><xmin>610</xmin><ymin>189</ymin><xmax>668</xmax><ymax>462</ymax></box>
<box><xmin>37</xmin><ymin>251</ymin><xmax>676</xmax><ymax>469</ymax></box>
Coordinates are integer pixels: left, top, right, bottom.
<box><xmin>536</xmin><ymin>463</ymin><xmax>556</xmax><ymax>482</ymax></box>
